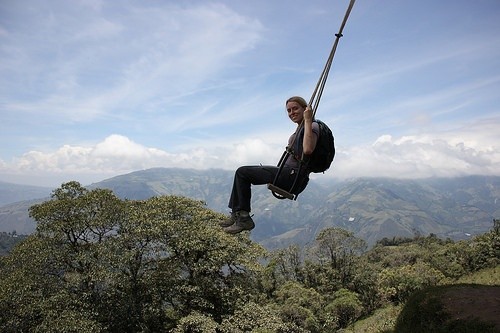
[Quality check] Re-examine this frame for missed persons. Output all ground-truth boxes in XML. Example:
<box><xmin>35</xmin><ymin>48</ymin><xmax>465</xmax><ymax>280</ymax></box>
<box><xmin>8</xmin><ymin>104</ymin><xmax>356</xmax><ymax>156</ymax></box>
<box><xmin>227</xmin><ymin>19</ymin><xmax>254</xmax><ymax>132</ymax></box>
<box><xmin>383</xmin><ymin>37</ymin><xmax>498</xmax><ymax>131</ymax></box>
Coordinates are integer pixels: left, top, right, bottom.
<box><xmin>217</xmin><ymin>96</ymin><xmax>321</xmax><ymax>235</ymax></box>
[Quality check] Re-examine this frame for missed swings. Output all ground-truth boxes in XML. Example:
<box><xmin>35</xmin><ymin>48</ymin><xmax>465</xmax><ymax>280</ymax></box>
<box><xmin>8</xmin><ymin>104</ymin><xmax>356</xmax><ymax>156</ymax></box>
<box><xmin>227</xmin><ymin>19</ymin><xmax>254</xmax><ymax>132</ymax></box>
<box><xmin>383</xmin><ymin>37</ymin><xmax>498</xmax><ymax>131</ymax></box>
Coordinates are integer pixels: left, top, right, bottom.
<box><xmin>267</xmin><ymin>0</ymin><xmax>355</xmax><ymax>200</ymax></box>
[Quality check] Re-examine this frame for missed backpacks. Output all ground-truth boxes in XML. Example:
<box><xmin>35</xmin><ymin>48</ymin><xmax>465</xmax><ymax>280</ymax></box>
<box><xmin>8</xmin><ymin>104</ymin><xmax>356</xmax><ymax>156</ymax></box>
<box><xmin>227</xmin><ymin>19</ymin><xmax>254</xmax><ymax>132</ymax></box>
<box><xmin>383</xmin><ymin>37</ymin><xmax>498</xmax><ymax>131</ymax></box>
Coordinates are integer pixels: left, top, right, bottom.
<box><xmin>308</xmin><ymin>118</ymin><xmax>335</xmax><ymax>173</ymax></box>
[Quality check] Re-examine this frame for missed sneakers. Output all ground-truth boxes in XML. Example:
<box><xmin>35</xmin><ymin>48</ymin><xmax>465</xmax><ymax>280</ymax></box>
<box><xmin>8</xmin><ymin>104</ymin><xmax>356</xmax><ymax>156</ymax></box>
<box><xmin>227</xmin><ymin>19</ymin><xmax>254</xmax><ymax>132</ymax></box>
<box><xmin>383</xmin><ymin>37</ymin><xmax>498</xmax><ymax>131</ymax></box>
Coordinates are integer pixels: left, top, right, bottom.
<box><xmin>218</xmin><ymin>211</ymin><xmax>237</xmax><ymax>226</ymax></box>
<box><xmin>224</xmin><ymin>213</ymin><xmax>255</xmax><ymax>233</ymax></box>
